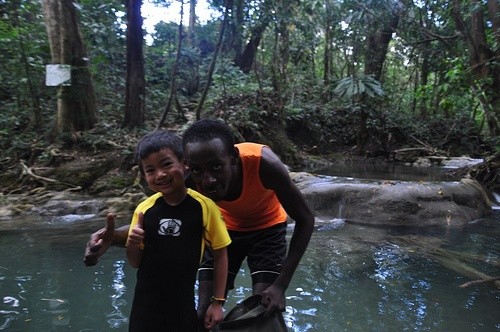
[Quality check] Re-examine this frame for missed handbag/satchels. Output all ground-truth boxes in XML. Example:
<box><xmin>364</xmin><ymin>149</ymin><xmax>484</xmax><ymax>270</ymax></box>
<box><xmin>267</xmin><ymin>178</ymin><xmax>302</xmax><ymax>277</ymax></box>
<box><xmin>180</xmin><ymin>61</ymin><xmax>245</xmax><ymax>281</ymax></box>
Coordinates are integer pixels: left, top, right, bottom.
<box><xmin>219</xmin><ymin>295</ymin><xmax>287</xmax><ymax>332</ymax></box>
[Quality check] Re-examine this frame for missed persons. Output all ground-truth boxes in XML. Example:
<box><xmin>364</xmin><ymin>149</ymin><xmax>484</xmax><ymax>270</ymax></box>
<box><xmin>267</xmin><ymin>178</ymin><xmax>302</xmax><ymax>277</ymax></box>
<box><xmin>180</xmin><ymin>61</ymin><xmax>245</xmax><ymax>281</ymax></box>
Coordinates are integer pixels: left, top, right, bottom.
<box><xmin>83</xmin><ymin>119</ymin><xmax>314</xmax><ymax>325</ymax></box>
<box><xmin>125</xmin><ymin>130</ymin><xmax>232</xmax><ymax>332</ymax></box>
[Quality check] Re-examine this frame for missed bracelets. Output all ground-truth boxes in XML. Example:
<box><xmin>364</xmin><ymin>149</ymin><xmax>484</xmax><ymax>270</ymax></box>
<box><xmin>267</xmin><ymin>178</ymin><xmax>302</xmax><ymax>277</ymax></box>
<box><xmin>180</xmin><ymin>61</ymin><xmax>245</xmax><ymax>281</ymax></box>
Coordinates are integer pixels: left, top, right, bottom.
<box><xmin>211</xmin><ymin>296</ymin><xmax>225</xmax><ymax>306</ymax></box>
